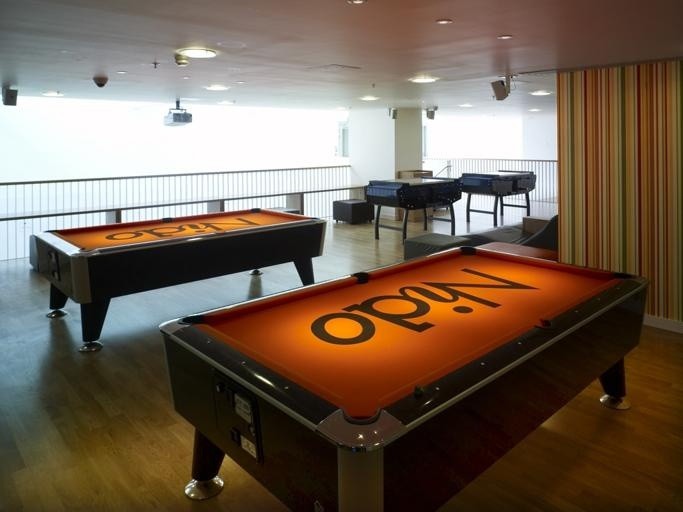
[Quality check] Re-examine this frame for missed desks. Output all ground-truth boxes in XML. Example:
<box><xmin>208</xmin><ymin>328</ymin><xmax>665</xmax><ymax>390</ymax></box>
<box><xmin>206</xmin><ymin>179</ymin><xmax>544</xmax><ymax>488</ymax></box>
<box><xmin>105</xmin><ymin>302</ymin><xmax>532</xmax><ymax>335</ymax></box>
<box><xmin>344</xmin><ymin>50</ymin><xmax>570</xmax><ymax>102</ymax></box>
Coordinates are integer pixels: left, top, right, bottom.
<box><xmin>158</xmin><ymin>244</ymin><xmax>648</xmax><ymax>512</ymax></box>
<box><xmin>30</xmin><ymin>208</ymin><xmax>329</xmax><ymax>354</ymax></box>
<box><xmin>363</xmin><ymin>169</ymin><xmax>537</xmax><ymax>243</ymax></box>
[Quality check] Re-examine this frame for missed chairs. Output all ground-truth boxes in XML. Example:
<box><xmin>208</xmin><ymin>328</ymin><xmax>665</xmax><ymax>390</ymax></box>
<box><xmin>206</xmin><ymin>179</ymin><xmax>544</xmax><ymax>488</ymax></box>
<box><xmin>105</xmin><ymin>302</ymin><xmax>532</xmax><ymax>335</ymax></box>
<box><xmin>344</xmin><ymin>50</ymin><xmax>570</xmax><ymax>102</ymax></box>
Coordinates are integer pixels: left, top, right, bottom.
<box><xmin>460</xmin><ymin>213</ymin><xmax>559</xmax><ymax>255</ymax></box>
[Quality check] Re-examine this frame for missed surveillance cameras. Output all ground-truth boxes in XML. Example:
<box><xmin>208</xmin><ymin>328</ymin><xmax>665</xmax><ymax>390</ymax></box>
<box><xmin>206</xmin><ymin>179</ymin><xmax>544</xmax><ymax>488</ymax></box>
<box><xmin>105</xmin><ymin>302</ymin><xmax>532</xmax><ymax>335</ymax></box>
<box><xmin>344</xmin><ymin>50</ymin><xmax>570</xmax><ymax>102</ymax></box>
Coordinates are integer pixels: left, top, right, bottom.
<box><xmin>93</xmin><ymin>77</ymin><xmax>108</xmax><ymax>87</ymax></box>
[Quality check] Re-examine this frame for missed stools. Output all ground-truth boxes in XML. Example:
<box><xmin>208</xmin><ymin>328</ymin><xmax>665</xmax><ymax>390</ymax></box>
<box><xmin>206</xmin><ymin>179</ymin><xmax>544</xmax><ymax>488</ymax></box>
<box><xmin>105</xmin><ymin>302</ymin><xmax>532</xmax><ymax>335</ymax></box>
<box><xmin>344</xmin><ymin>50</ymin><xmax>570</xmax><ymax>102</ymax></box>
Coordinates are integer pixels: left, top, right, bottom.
<box><xmin>402</xmin><ymin>232</ymin><xmax>471</xmax><ymax>261</ymax></box>
<box><xmin>332</xmin><ymin>198</ymin><xmax>375</xmax><ymax>226</ymax></box>
